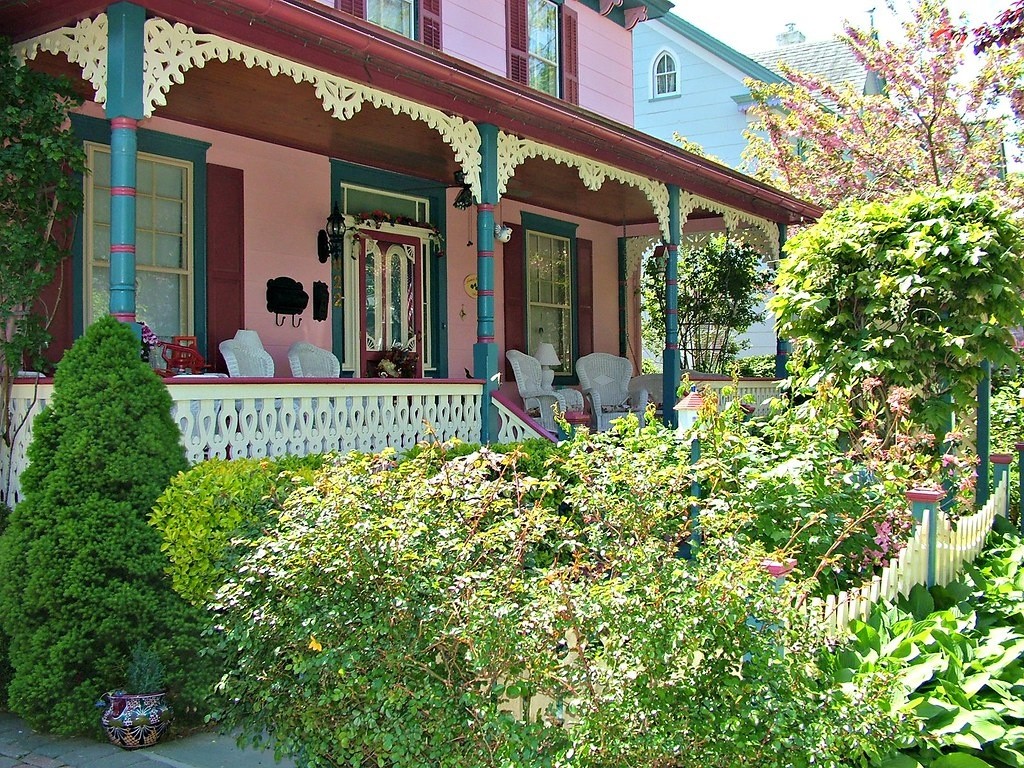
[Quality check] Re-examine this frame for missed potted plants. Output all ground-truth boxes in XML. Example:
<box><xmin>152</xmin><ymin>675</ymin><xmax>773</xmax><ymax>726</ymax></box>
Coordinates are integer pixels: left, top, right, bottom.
<box><xmin>101</xmin><ymin>639</ymin><xmax>175</xmax><ymax>748</ymax></box>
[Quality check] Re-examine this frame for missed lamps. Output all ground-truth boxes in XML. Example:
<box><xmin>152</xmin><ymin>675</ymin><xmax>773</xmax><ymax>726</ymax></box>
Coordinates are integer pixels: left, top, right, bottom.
<box><xmin>533</xmin><ymin>343</ymin><xmax>562</xmax><ymax>391</ymax></box>
<box><xmin>234</xmin><ymin>330</ymin><xmax>266</xmax><ymax>351</ymax></box>
<box><xmin>317</xmin><ymin>201</ymin><xmax>346</xmax><ymax>264</ymax></box>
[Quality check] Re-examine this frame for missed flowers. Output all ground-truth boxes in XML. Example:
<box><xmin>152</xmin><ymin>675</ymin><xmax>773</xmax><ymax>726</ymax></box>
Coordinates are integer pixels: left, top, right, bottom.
<box><xmin>95</xmin><ymin>688</ymin><xmax>125</xmax><ymax>709</ymax></box>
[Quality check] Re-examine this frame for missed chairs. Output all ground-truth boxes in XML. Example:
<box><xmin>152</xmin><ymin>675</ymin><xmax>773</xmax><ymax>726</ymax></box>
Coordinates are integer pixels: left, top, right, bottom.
<box><xmin>575</xmin><ymin>352</ymin><xmax>649</xmax><ymax>433</ymax></box>
<box><xmin>288</xmin><ymin>341</ymin><xmax>388</xmax><ymax>433</ymax></box>
<box><xmin>506</xmin><ymin>349</ymin><xmax>585</xmax><ymax>435</ymax></box>
<box><xmin>219</xmin><ymin>339</ymin><xmax>333</xmax><ymax>434</ymax></box>
